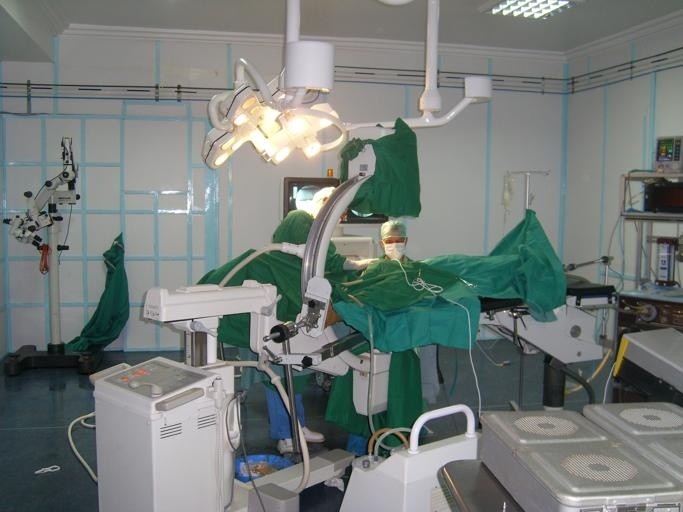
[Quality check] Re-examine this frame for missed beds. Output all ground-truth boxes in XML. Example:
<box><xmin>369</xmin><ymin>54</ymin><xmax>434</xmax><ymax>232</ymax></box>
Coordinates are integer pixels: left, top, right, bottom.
<box><xmin>186</xmin><ymin>276</ymin><xmax>615</xmax><ymax>409</ymax></box>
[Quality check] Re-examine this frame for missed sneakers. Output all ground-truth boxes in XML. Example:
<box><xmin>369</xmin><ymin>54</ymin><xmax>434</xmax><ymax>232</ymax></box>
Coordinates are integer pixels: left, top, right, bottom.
<box><xmin>277</xmin><ymin>438</ymin><xmax>292</xmax><ymax>455</ymax></box>
<box><xmin>302</xmin><ymin>425</ymin><xmax>326</xmax><ymax>444</ymax></box>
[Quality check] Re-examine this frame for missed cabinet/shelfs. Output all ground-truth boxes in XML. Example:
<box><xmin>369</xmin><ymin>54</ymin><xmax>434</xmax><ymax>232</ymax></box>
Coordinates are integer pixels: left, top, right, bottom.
<box><xmin>613</xmin><ymin>291</ymin><xmax>683</xmax><ymax>407</ymax></box>
<box><xmin>619</xmin><ymin>173</ymin><xmax>683</xmax><ymax>221</ymax></box>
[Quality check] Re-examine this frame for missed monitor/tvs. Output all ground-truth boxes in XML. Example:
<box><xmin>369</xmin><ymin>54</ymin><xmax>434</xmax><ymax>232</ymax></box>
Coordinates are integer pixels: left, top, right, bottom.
<box><xmin>281</xmin><ymin>176</ymin><xmax>344</xmax><ymax>226</ymax></box>
<box><xmin>342</xmin><ymin>207</ymin><xmax>390</xmax><ymax>224</ymax></box>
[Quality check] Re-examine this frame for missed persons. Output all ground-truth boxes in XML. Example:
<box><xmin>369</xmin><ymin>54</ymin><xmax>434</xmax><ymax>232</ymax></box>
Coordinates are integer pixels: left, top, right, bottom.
<box><xmin>328</xmin><ymin>217</ymin><xmax>423</xmax><ymax>459</ymax></box>
<box><xmin>259</xmin><ymin>182</ymin><xmax>381</xmax><ymax>458</ymax></box>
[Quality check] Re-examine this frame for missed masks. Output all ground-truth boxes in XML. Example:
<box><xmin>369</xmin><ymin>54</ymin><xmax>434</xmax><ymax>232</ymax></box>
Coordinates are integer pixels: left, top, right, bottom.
<box><xmin>383</xmin><ymin>242</ymin><xmax>407</xmax><ymax>260</ymax></box>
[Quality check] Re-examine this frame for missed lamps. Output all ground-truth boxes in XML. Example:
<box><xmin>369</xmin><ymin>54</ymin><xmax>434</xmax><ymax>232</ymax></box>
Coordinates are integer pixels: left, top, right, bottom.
<box><xmin>201</xmin><ymin>0</ymin><xmax>492</xmax><ymax>169</ymax></box>
<box><xmin>480</xmin><ymin>0</ymin><xmax>576</xmax><ymax>24</ymax></box>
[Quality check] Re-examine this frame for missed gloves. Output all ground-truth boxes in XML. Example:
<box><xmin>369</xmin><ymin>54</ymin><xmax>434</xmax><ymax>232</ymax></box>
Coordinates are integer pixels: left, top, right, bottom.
<box><xmin>342</xmin><ymin>257</ymin><xmax>379</xmax><ymax>271</ymax></box>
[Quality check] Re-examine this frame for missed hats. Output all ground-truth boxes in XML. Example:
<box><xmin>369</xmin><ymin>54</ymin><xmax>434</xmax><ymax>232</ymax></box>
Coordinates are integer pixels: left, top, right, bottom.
<box><xmin>380</xmin><ymin>219</ymin><xmax>407</xmax><ymax>241</ymax></box>
